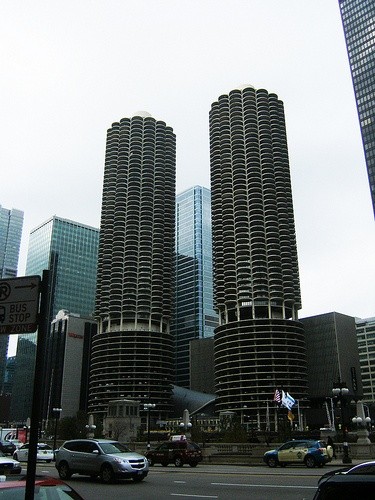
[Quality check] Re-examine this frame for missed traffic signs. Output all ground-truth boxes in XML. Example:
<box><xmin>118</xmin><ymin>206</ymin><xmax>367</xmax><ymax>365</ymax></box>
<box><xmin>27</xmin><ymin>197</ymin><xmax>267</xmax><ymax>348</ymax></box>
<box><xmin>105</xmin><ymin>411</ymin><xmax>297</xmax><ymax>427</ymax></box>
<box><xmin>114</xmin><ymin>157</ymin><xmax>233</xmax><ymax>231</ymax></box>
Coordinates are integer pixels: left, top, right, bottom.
<box><xmin>1</xmin><ymin>274</ymin><xmax>40</xmax><ymax>334</ymax></box>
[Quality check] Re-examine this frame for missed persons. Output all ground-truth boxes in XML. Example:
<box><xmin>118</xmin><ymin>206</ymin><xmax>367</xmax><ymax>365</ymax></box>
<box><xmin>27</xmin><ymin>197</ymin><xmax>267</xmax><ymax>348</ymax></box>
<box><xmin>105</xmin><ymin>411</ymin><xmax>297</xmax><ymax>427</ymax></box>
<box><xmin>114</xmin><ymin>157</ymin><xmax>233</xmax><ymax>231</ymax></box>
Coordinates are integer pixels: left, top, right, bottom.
<box><xmin>327</xmin><ymin>436</ymin><xmax>337</xmax><ymax>460</ymax></box>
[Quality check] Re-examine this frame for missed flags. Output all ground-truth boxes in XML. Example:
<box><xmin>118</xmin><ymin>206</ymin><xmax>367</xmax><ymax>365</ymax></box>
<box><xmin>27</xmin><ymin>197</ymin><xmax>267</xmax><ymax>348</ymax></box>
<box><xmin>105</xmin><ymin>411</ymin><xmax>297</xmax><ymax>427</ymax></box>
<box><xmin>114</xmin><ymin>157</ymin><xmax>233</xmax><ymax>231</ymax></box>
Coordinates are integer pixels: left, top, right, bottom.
<box><xmin>274</xmin><ymin>389</ymin><xmax>280</xmax><ymax>402</ymax></box>
<box><xmin>285</xmin><ymin>392</ymin><xmax>295</xmax><ymax>408</ymax></box>
<box><xmin>281</xmin><ymin>390</ymin><xmax>291</xmax><ymax>410</ymax></box>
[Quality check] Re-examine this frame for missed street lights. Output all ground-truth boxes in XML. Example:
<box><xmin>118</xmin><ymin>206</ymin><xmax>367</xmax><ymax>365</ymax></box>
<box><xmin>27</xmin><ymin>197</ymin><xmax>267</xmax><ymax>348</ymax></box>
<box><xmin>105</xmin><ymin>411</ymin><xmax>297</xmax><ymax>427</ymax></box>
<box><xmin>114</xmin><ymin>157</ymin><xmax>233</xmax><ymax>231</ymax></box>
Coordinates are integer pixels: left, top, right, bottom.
<box><xmin>329</xmin><ymin>378</ymin><xmax>353</xmax><ymax>463</ymax></box>
<box><xmin>143</xmin><ymin>403</ymin><xmax>156</xmax><ymax>442</ymax></box>
<box><xmin>52</xmin><ymin>408</ymin><xmax>63</xmax><ymax>442</ymax></box>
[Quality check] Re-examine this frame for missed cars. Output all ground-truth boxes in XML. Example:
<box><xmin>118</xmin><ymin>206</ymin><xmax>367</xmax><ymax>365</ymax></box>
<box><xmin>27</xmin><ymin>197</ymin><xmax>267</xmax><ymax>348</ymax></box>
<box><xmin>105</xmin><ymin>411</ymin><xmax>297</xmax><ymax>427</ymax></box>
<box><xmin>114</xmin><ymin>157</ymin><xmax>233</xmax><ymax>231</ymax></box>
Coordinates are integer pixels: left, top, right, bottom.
<box><xmin>13</xmin><ymin>443</ymin><xmax>54</xmax><ymax>463</ymax></box>
<box><xmin>0</xmin><ymin>444</ymin><xmax>22</xmax><ymax>475</ymax></box>
<box><xmin>0</xmin><ymin>439</ymin><xmax>16</xmax><ymax>455</ymax></box>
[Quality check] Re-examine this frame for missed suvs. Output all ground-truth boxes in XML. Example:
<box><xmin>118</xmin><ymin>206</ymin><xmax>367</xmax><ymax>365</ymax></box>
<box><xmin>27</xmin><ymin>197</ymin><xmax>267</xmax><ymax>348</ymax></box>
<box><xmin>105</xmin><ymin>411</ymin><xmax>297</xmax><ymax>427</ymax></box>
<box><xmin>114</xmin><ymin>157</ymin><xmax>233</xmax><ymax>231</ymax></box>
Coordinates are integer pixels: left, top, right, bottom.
<box><xmin>143</xmin><ymin>439</ymin><xmax>202</xmax><ymax>468</ymax></box>
<box><xmin>0</xmin><ymin>474</ymin><xmax>84</xmax><ymax>500</ymax></box>
<box><xmin>313</xmin><ymin>459</ymin><xmax>375</xmax><ymax>500</ymax></box>
<box><xmin>264</xmin><ymin>438</ymin><xmax>334</xmax><ymax>467</ymax></box>
<box><xmin>56</xmin><ymin>439</ymin><xmax>149</xmax><ymax>482</ymax></box>
